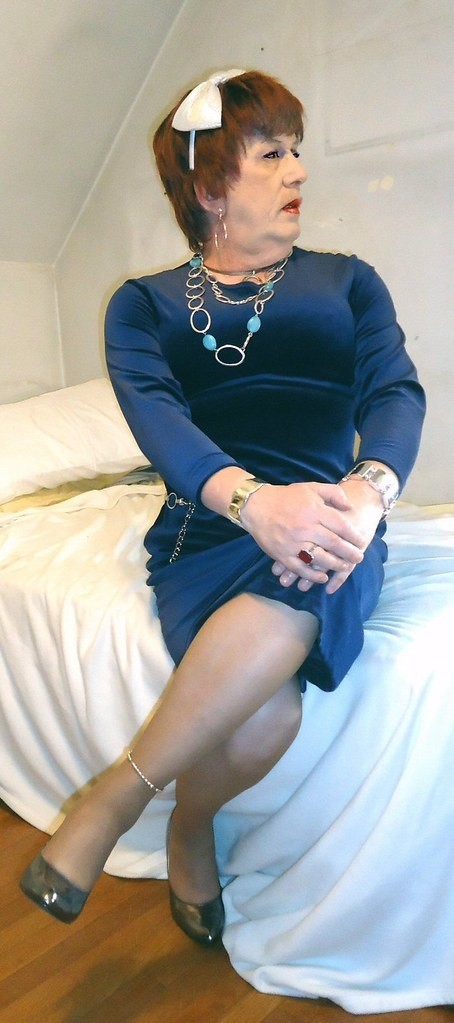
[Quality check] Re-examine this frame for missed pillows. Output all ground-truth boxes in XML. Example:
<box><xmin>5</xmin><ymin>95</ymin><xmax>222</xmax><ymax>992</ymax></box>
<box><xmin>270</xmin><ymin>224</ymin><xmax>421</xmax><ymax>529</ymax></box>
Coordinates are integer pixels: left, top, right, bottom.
<box><xmin>1</xmin><ymin>377</ymin><xmax>166</xmax><ymax>505</ymax></box>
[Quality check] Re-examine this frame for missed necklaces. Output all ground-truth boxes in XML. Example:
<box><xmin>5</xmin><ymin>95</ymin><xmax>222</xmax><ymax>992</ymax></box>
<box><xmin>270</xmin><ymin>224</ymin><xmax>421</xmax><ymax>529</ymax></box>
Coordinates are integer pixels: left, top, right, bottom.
<box><xmin>186</xmin><ymin>243</ymin><xmax>293</xmax><ymax>367</ymax></box>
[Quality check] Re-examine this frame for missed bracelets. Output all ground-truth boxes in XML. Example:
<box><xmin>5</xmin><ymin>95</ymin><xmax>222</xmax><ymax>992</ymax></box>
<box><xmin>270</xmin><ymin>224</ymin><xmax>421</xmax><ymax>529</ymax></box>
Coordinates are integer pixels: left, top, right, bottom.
<box><xmin>338</xmin><ymin>462</ymin><xmax>398</xmax><ymax>522</ymax></box>
<box><xmin>226</xmin><ymin>478</ymin><xmax>271</xmax><ymax>526</ymax></box>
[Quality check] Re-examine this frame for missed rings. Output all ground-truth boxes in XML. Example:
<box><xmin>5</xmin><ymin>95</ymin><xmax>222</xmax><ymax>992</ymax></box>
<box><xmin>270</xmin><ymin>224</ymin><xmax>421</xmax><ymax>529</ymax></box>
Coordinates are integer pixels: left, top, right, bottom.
<box><xmin>296</xmin><ymin>545</ymin><xmax>320</xmax><ymax>566</ymax></box>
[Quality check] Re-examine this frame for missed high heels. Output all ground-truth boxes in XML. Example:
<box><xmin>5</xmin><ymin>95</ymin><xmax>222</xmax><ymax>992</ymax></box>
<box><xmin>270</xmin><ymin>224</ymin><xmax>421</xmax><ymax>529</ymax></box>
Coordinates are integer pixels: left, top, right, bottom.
<box><xmin>165</xmin><ymin>804</ymin><xmax>225</xmax><ymax>945</ymax></box>
<box><xmin>19</xmin><ymin>837</ymin><xmax>91</xmax><ymax>924</ymax></box>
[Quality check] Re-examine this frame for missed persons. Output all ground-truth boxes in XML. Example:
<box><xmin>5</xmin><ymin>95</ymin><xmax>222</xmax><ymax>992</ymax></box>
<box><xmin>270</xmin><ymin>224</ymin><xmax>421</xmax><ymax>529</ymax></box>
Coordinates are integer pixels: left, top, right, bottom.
<box><xmin>18</xmin><ymin>70</ymin><xmax>427</xmax><ymax>949</ymax></box>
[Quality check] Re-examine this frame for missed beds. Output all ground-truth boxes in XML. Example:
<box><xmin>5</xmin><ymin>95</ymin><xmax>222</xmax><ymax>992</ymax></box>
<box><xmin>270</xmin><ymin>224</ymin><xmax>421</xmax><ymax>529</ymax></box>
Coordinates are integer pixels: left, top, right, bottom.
<box><xmin>0</xmin><ymin>475</ymin><xmax>452</xmax><ymax>1015</ymax></box>
